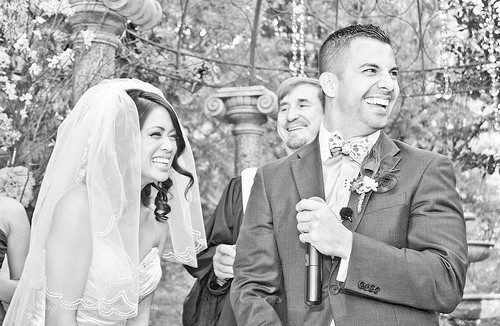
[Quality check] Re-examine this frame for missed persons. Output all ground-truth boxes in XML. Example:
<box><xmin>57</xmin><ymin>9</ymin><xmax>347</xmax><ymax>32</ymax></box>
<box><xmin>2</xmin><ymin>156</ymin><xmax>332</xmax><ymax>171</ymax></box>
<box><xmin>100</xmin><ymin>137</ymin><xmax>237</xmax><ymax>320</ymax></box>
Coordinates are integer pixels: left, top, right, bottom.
<box><xmin>230</xmin><ymin>24</ymin><xmax>468</xmax><ymax>326</ymax></box>
<box><xmin>0</xmin><ymin>78</ymin><xmax>209</xmax><ymax>326</ymax></box>
<box><xmin>0</xmin><ymin>195</ymin><xmax>30</xmax><ymax>326</ymax></box>
<box><xmin>183</xmin><ymin>77</ymin><xmax>324</xmax><ymax>326</ymax></box>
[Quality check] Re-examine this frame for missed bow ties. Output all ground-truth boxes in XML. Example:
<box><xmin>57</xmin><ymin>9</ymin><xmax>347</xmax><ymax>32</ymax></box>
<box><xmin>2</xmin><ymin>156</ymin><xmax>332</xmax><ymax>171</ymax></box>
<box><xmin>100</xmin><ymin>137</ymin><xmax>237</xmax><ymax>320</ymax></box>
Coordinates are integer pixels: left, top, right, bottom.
<box><xmin>329</xmin><ymin>131</ymin><xmax>369</xmax><ymax>164</ymax></box>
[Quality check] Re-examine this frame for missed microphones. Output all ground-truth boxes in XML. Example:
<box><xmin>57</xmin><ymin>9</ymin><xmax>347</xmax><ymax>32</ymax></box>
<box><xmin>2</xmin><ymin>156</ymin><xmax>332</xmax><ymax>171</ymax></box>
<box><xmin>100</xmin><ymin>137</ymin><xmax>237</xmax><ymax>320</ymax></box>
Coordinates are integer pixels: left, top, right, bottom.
<box><xmin>304</xmin><ymin>197</ymin><xmax>325</xmax><ymax>306</ymax></box>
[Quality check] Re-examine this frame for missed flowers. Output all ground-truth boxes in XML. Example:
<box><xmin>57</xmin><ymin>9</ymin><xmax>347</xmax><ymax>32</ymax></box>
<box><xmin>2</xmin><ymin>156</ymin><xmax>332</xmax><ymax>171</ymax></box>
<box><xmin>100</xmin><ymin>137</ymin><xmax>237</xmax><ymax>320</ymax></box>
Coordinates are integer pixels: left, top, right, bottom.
<box><xmin>349</xmin><ymin>146</ymin><xmax>400</xmax><ymax>214</ymax></box>
